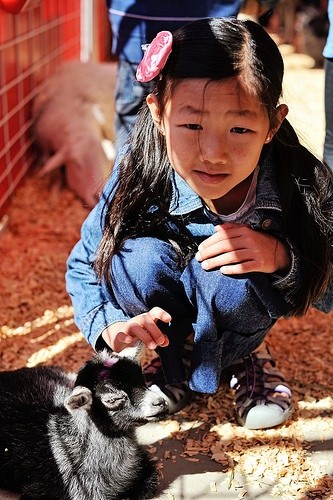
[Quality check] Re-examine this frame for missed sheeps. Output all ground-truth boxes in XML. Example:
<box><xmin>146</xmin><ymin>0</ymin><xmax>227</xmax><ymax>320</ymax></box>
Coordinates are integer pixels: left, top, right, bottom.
<box><xmin>0</xmin><ymin>347</ymin><xmax>169</xmax><ymax>500</ymax></box>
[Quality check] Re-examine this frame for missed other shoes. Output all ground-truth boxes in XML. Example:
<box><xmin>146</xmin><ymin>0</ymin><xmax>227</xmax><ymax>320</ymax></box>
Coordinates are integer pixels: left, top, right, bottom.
<box><xmin>146</xmin><ymin>374</ymin><xmax>189</xmax><ymax>410</ymax></box>
<box><xmin>224</xmin><ymin>341</ymin><xmax>295</xmax><ymax>431</ymax></box>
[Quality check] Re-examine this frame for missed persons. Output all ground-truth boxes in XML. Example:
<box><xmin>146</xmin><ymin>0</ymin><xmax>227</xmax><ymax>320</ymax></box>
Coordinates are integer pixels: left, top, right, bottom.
<box><xmin>107</xmin><ymin>0</ymin><xmax>244</xmax><ymax>163</ymax></box>
<box><xmin>321</xmin><ymin>0</ymin><xmax>333</xmax><ymax>172</ymax></box>
<box><xmin>64</xmin><ymin>18</ymin><xmax>333</xmax><ymax>429</ymax></box>
